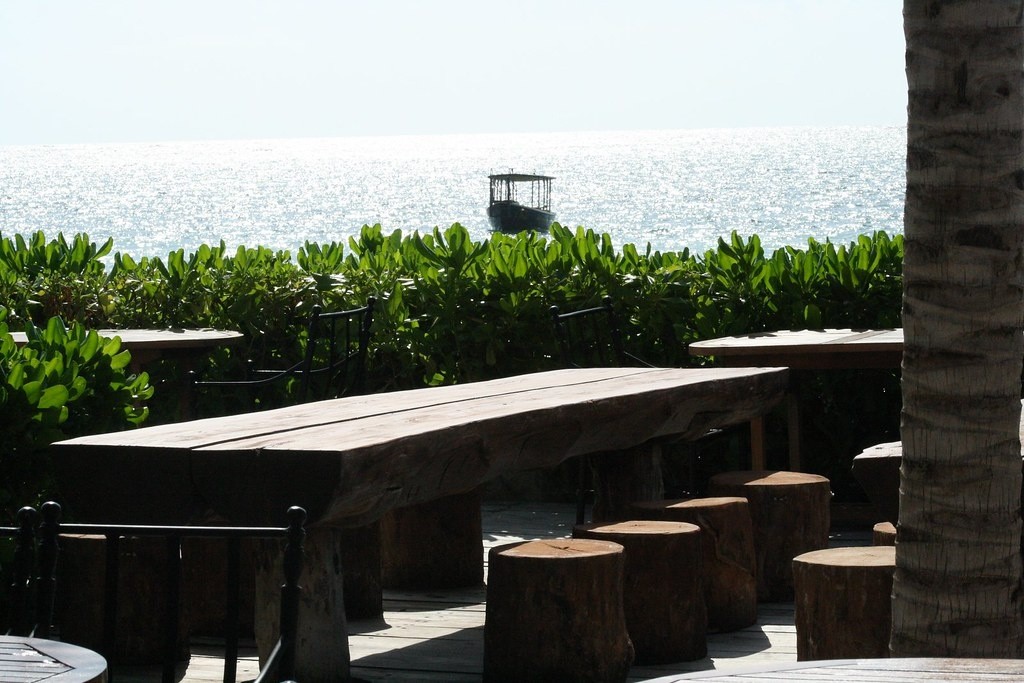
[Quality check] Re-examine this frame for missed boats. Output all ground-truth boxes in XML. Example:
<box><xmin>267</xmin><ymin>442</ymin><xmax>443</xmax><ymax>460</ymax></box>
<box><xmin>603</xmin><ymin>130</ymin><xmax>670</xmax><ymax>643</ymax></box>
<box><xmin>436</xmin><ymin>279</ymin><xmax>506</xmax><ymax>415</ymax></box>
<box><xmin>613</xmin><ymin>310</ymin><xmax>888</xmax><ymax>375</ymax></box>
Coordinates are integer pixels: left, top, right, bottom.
<box><xmin>486</xmin><ymin>166</ymin><xmax>556</xmax><ymax>235</ymax></box>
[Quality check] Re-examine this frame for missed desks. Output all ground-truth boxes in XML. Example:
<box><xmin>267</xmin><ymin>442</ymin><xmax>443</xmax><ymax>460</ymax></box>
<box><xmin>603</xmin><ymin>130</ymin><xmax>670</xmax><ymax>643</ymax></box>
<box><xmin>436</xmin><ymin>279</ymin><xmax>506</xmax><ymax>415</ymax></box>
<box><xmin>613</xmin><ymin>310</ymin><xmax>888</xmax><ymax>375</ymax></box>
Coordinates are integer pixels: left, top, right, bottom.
<box><xmin>689</xmin><ymin>325</ymin><xmax>905</xmax><ymax>467</ymax></box>
<box><xmin>49</xmin><ymin>365</ymin><xmax>795</xmax><ymax>683</ymax></box>
<box><xmin>0</xmin><ymin>634</ymin><xmax>109</xmax><ymax>683</ymax></box>
<box><xmin>6</xmin><ymin>328</ymin><xmax>244</xmax><ymax>372</ymax></box>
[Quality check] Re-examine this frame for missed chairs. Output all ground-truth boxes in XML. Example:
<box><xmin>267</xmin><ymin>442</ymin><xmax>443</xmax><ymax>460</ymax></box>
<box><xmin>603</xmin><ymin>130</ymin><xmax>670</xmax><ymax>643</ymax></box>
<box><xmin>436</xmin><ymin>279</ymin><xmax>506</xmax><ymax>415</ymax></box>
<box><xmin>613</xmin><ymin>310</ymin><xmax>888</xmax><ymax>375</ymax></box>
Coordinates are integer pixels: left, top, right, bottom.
<box><xmin>0</xmin><ymin>503</ymin><xmax>308</xmax><ymax>683</ymax></box>
<box><xmin>192</xmin><ymin>295</ymin><xmax>377</xmax><ymax>415</ymax></box>
<box><xmin>550</xmin><ymin>295</ymin><xmax>657</xmax><ymax>368</ymax></box>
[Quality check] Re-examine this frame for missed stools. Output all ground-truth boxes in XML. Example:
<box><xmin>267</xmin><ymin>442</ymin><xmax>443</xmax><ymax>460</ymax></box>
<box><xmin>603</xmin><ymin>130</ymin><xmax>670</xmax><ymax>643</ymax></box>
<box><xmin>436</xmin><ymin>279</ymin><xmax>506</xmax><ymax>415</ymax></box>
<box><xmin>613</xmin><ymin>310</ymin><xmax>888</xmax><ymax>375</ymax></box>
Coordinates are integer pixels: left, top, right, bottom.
<box><xmin>342</xmin><ymin>433</ymin><xmax>904</xmax><ymax>678</ymax></box>
